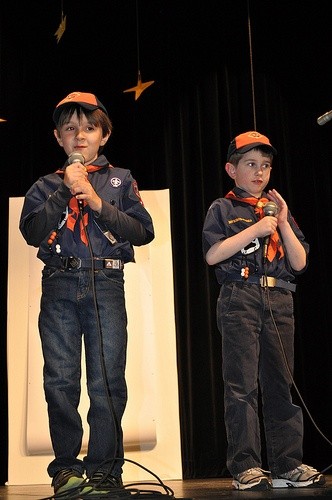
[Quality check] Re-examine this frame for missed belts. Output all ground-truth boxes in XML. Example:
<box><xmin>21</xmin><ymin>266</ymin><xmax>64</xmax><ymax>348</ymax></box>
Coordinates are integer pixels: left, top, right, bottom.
<box><xmin>224</xmin><ymin>274</ymin><xmax>296</xmax><ymax>292</ymax></box>
<box><xmin>46</xmin><ymin>256</ymin><xmax>124</xmax><ymax>271</ymax></box>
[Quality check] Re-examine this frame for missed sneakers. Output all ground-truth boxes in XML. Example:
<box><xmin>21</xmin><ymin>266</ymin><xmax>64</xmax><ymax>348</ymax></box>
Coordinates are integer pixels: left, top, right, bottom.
<box><xmin>232</xmin><ymin>467</ymin><xmax>271</xmax><ymax>490</ymax></box>
<box><xmin>80</xmin><ymin>468</ymin><xmax>123</xmax><ymax>494</ymax></box>
<box><xmin>51</xmin><ymin>468</ymin><xmax>85</xmax><ymax>494</ymax></box>
<box><xmin>271</xmin><ymin>463</ymin><xmax>326</xmax><ymax>488</ymax></box>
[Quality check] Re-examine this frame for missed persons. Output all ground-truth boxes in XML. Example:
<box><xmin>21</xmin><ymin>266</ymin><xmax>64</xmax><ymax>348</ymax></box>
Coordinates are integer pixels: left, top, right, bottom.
<box><xmin>201</xmin><ymin>130</ymin><xmax>325</xmax><ymax>492</ymax></box>
<box><xmin>18</xmin><ymin>92</ymin><xmax>155</xmax><ymax>496</ymax></box>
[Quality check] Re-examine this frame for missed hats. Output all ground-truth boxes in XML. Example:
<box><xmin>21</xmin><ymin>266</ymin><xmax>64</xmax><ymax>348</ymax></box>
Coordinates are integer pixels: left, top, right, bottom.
<box><xmin>227</xmin><ymin>131</ymin><xmax>277</xmax><ymax>160</ymax></box>
<box><xmin>53</xmin><ymin>92</ymin><xmax>108</xmax><ymax>127</ymax></box>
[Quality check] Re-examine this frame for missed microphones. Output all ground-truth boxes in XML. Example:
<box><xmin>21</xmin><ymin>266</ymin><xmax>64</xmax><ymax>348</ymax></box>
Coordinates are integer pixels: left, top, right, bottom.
<box><xmin>262</xmin><ymin>202</ymin><xmax>277</xmax><ymax>265</ymax></box>
<box><xmin>68</xmin><ymin>151</ymin><xmax>84</xmax><ymax>211</ymax></box>
<box><xmin>317</xmin><ymin>110</ymin><xmax>332</xmax><ymax>126</ymax></box>
